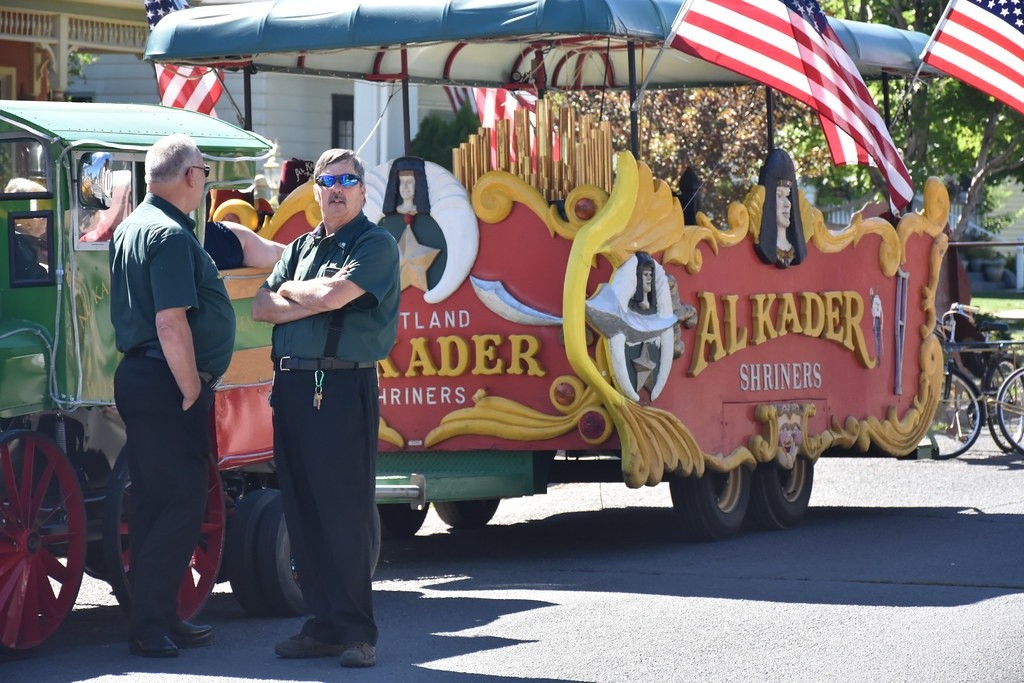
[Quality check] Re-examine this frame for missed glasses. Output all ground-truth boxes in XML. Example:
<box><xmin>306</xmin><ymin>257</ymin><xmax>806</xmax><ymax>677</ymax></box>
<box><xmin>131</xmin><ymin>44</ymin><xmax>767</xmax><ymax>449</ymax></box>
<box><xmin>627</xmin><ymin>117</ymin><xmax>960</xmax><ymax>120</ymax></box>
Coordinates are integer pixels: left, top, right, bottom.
<box><xmin>185</xmin><ymin>165</ymin><xmax>210</xmax><ymax>177</ymax></box>
<box><xmin>315</xmin><ymin>173</ymin><xmax>362</xmax><ymax>189</ymax></box>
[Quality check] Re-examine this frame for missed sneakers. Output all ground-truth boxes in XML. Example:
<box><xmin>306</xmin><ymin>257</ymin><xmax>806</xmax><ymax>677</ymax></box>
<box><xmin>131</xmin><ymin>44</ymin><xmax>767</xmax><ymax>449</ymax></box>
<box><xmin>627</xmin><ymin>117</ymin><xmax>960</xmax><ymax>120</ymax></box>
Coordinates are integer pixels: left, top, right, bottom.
<box><xmin>340</xmin><ymin>639</ymin><xmax>376</xmax><ymax>666</ymax></box>
<box><xmin>274</xmin><ymin>631</ymin><xmax>344</xmax><ymax>658</ymax></box>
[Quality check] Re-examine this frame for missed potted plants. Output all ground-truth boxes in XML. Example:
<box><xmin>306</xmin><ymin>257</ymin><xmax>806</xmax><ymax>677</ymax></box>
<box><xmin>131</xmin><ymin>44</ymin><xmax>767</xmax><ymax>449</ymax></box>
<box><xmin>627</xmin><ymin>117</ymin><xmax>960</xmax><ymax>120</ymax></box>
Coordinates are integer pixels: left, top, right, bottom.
<box><xmin>958</xmin><ymin>228</ymin><xmax>1006</xmax><ymax>281</ymax></box>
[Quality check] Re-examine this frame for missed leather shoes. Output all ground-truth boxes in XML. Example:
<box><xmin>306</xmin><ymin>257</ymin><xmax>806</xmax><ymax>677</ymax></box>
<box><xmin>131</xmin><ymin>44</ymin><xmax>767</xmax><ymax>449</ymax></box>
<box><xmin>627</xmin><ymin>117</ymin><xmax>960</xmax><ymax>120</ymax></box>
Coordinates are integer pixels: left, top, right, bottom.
<box><xmin>126</xmin><ymin>633</ymin><xmax>180</xmax><ymax>657</ymax></box>
<box><xmin>167</xmin><ymin>619</ymin><xmax>215</xmax><ymax>647</ymax></box>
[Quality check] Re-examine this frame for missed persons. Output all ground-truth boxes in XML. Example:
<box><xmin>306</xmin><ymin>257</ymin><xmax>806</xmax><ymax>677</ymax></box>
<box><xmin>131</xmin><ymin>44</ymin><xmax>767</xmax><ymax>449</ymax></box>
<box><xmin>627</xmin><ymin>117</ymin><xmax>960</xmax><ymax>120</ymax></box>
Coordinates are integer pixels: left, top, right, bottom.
<box><xmin>381</xmin><ymin>156</ymin><xmax>430</xmax><ymax>216</ymax></box>
<box><xmin>627</xmin><ymin>251</ymin><xmax>659</xmax><ymax>315</ymax></box>
<box><xmin>755</xmin><ymin>148</ymin><xmax>808</xmax><ymax>270</ymax></box>
<box><xmin>251</xmin><ymin>148</ymin><xmax>401</xmax><ymax>666</ymax></box>
<box><xmin>204</xmin><ymin>184</ymin><xmax>289</xmax><ymax>270</ymax></box>
<box><xmin>3</xmin><ymin>177</ymin><xmax>50</xmax><ymax>284</ymax></box>
<box><xmin>110</xmin><ymin>133</ymin><xmax>236</xmax><ymax>658</ymax></box>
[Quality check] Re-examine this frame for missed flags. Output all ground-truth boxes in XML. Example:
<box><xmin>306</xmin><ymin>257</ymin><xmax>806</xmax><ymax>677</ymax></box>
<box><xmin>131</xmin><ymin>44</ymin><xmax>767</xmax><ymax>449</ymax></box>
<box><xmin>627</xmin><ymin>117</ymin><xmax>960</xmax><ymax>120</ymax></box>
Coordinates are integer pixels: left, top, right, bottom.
<box><xmin>144</xmin><ymin>0</ymin><xmax>226</xmax><ymax>121</ymax></box>
<box><xmin>471</xmin><ymin>86</ymin><xmax>566</xmax><ymax>173</ymax></box>
<box><xmin>670</xmin><ymin>0</ymin><xmax>916</xmax><ymax>218</ymax></box>
<box><xmin>919</xmin><ymin>0</ymin><xmax>1024</xmax><ymax>113</ymax></box>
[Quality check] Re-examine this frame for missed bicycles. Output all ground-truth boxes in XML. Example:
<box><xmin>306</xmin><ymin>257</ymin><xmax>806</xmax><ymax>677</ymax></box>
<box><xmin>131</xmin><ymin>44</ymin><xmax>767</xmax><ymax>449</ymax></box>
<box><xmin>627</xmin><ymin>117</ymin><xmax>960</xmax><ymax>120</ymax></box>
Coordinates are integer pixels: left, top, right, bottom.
<box><xmin>917</xmin><ymin>301</ymin><xmax>1024</xmax><ymax>459</ymax></box>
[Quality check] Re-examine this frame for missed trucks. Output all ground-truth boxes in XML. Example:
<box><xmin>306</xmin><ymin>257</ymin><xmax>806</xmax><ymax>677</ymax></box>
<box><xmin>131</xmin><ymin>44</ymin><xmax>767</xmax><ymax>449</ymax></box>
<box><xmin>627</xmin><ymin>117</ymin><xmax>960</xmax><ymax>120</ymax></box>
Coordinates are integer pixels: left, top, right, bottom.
<box><xmin>0</xmin><ymin>0</ymin><xmax>949</xmax><ymax>666</ymax></box>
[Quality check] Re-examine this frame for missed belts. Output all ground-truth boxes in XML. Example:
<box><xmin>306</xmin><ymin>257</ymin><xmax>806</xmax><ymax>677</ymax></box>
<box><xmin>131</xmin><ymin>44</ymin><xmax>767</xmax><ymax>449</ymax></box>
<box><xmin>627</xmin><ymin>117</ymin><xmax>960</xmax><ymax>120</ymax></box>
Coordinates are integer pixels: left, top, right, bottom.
<box><xmin>272</xmin><ymin>356</ymin><xmax>379</xmax><ymax>372</ymax></box>
<box><xmin>127</xmin><ymin>347</ymin><xmax>221</xmax><ymax>389</ymax></box>
